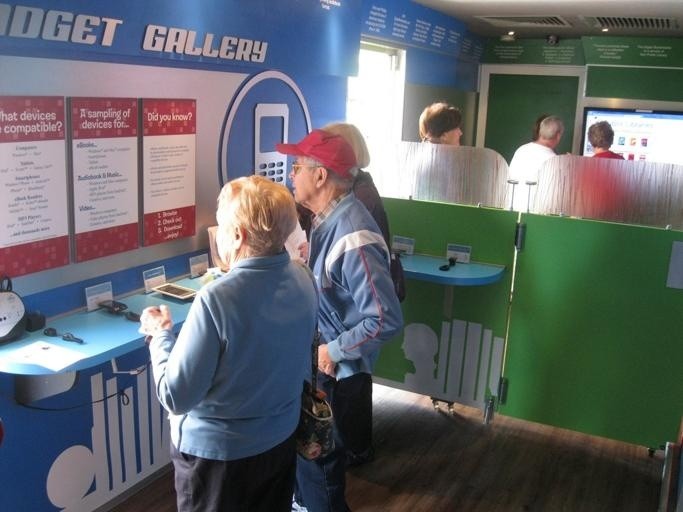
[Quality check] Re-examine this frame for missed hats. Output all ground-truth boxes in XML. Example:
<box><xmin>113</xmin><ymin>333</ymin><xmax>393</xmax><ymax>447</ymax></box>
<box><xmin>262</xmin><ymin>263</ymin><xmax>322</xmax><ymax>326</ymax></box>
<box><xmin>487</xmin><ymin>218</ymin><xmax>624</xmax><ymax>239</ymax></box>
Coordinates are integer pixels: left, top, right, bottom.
<box><xmin>275</xmin><ymin>129</ymin><xmax>356</xmax><ymax>177</ymax></box>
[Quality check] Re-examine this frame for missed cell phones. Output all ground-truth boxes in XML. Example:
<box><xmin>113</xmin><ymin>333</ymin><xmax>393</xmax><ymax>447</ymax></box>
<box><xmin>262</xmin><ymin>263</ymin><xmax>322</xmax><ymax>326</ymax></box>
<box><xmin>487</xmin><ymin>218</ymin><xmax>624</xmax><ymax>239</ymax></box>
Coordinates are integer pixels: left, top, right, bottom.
<box><xmin>98</xmin><ymin>299</ymin><xmax>128</xmax><ymax>312</ymax></box>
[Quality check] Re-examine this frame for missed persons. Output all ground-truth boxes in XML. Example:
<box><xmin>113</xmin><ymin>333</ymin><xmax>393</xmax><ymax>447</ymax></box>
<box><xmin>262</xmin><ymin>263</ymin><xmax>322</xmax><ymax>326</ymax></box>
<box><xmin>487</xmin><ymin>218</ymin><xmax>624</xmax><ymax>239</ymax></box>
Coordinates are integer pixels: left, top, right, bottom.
<box><xmin>292</xmin><ymin>121</ymin><xmax>391</xmax><ymax>255</ymax></box>
<box><xmin>587</xmin><ymin>119</ymin><xmax>626</xmax><ymax>160</ymax></box>
<box><xmin>532</xmin><ymin>113</ymin><xmax>551</xmax><ymax>139</ymax></box>
<box><xmin>418</xmin><ymin>101</ymin><xmax>463</xmax><ymax>146</ymax></box>
<box><xmin>273</xmin><ymin>131</ymin><xmax>404</xmax><ymax>512</ymax></box>
<box><xmin>508</xmin><ymin>115</ymin><xmax>567</xmax><ymax>215</ymax></box>
<box><xmin>137</xmin><ymin>169</ymin><xmax>323</xmax><ymax>512</ymax></box>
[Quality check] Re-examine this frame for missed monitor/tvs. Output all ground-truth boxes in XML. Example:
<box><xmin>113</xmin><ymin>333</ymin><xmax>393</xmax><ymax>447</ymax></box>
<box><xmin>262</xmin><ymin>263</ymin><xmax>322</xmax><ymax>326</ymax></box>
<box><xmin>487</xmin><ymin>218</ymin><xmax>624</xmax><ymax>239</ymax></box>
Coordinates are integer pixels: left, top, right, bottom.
<box><xmin>580</xmin><ymin>107</ymin><xmax>683</xmax><ymax>165</ymax></box>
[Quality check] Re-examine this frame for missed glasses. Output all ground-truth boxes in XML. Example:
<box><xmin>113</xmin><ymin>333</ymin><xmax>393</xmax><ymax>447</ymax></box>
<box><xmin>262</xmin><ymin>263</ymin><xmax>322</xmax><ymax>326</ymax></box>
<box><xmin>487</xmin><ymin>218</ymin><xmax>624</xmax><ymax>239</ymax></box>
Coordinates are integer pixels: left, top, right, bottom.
<box><xmin>292</xmin><ymin>161</ymin><xmax>323</xmax><ymax>174</ymax></box>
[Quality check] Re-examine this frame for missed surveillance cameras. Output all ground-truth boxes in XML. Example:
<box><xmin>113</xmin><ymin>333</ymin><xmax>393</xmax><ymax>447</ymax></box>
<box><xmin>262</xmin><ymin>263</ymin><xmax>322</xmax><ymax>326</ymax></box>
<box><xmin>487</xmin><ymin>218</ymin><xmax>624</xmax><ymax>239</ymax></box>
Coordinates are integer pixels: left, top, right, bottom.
<box><xmin>547</xmin><ymin>34</ymin><xmax>559</xmax><ymax>46</ymax></box>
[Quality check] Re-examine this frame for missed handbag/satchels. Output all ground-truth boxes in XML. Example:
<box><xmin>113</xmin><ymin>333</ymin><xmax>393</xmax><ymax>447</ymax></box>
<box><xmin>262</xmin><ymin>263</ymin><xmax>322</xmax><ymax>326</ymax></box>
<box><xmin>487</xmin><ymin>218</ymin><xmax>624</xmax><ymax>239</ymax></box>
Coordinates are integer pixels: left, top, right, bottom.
<box><xmin>391</xmin><ymin>252</ymin><xmax>405</xmax><ymax>302</ymax></box>
<box><xmin>296</xmin><ymin>379</ymin><xmax>336</xmax><ymax>461</ymax></box>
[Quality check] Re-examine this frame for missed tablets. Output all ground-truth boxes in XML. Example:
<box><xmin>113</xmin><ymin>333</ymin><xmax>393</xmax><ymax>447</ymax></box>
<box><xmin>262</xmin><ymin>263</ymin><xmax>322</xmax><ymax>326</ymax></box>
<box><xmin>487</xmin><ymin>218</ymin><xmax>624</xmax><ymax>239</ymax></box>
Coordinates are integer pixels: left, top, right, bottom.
<box><xmin>151</xmin><ymin>283</ymin><xmax>198</xmax><ymax>300</ymax></box>
